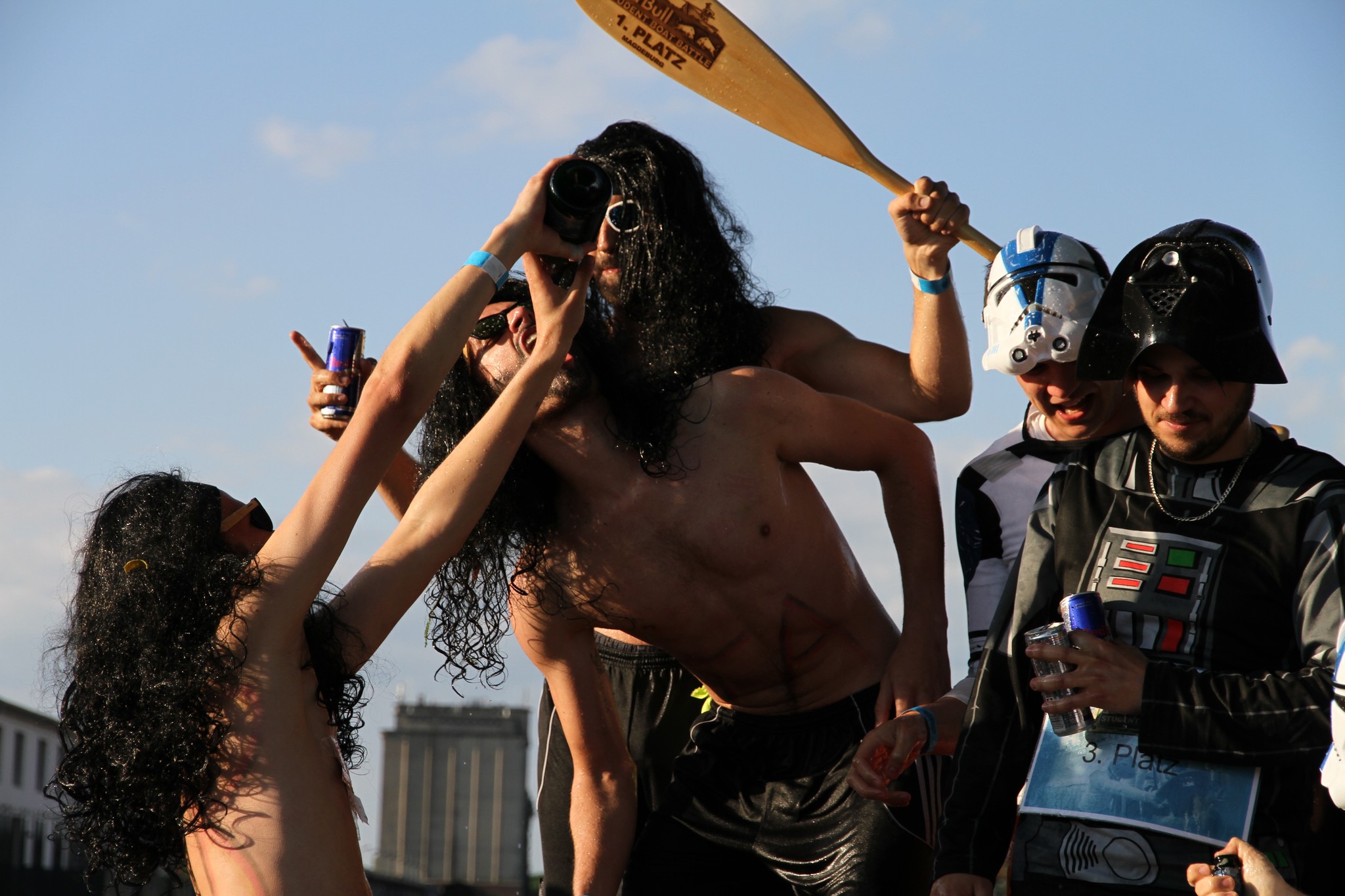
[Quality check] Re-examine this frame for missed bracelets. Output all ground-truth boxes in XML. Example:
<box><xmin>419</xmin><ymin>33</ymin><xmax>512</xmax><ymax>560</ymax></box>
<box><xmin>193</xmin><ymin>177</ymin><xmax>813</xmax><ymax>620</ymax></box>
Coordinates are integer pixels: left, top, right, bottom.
<box><xmin>909</xmin><ymin>257</ymin><xmax>954</xmax><ymax>294</ymax></box>
<box><xmin>461</xmin><ymin>251</ymin><xmax>510</xmax><ymax>291</ymax></box>
<box><xmin>899</xmin><ymin>706</ymin><xmax>940</xmax><ymax>753</ymax></box>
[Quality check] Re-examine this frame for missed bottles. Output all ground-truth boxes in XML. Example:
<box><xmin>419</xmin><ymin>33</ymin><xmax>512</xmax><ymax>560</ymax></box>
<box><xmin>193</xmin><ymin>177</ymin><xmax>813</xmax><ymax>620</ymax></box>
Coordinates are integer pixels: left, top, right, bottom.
<box><xmin>1215</xmin><ymin>854</ymin><xmax>1247</xmax><ymax>896</ymax></box>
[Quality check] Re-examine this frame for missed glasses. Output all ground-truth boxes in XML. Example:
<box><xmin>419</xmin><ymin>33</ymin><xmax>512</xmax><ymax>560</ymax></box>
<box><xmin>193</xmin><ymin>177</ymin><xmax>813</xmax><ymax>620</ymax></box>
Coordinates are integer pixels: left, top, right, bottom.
<box><xmin>462</xmin><ymin>301</ymin><xmax>533</xmax><ymax>375</ymax></box>
<box><xmin>220</xmin><ymin>497</ymin><xmax>273</xmax><ymax>535</ymax></box>
<box><xmin>605</xmin><ymin>199</ymin><xmax>646</xmax><ymax>234</ymax></box>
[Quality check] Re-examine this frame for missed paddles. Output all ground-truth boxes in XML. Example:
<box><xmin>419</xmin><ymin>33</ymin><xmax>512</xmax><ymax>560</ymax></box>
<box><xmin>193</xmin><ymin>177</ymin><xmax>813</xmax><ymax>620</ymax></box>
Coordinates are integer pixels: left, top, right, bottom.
<box><xmin>577</xmin><ymin>0</ymin><xmax>1002</xmax><ymax>264</ymax></box>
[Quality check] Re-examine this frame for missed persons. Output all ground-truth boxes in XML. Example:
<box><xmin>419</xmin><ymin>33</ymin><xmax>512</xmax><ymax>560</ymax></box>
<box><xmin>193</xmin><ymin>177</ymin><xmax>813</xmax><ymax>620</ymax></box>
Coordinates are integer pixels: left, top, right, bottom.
<box><xmin>44</xmin><ymin>153</ymin><xmax>596</xmax><ymax>896</ymax></box>
<box><xmin>289</xmin><ymin>121</ymin><xmax>1345</xmax><ymax>896</ymax></box>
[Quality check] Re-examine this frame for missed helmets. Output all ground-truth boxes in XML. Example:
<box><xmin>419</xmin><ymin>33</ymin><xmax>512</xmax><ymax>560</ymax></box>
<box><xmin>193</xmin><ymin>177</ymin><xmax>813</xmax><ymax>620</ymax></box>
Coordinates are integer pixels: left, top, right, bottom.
<box><xmin>981</xmin><ymin>224</ymin><xmax>1109</xmax><ymax>375</ymax></box>
<box><xmin>1076</xmin><ymin>218</ymin><xmax>1289</xmax><ymax>385</ymax></box>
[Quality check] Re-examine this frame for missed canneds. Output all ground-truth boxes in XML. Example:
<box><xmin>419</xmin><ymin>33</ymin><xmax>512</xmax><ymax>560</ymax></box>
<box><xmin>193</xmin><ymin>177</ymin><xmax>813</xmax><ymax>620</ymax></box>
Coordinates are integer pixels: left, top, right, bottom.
<box><xmin>1024</xmin><ymin>622</ymin><xmax>1094</xmax><ymax>737</ymax></box>
<box><xmin>1060</xmin><ymin>591</ymin><xmax>1114</xmax><ymax>649</ymax></box>
<box><xmin>320</xmin><ymin>319</ymin><xmax>365</xmax><ymax>420</ymax></box>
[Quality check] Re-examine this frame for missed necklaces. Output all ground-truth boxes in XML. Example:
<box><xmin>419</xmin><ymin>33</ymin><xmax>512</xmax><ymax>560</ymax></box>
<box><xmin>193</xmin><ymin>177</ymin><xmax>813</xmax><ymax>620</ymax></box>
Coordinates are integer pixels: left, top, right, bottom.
<box><xmin>1148</xmin><ymin>426</ymin><xmax>1261</xmax><ymax>522</ymax></box>
